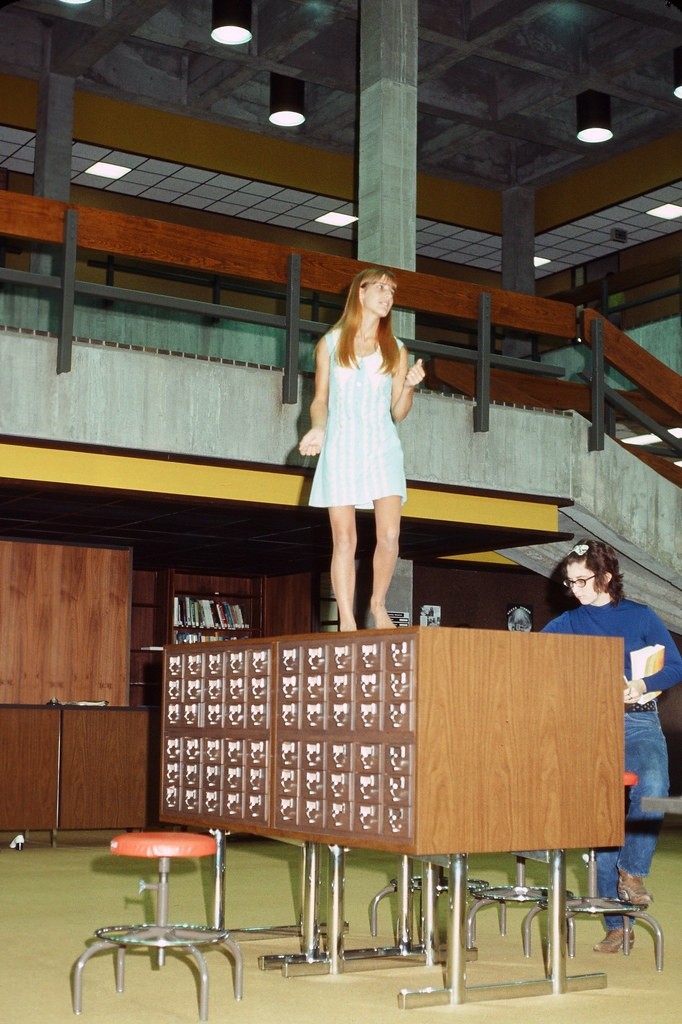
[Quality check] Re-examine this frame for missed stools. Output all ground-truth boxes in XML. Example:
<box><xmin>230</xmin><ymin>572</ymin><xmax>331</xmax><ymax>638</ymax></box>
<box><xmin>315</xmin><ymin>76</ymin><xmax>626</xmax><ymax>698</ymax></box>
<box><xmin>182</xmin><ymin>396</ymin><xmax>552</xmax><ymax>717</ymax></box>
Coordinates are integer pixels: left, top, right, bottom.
<box><xmin>370</xmin><ymin>770</ymin><xmax>664</xmax><ymax>975</ymax></box>
<box><xmin>66</xmin><ymin>831</ymin><xmax>242</xmax><ymax>1021</ymax></box>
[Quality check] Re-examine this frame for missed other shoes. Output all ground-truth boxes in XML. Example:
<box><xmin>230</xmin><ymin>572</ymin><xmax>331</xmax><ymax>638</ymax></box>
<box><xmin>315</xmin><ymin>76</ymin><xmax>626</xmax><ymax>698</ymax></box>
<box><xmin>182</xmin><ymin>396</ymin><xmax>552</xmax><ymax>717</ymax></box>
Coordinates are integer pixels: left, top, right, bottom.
<box><xmin>593</xmin><ymin>927</ymin><xmax>634</xmax><ymax>953</ymax></box>
<box><xmin>618</xmin><ymin>869</ymin><xmax>654</xmax><ymax>904</ymax></box>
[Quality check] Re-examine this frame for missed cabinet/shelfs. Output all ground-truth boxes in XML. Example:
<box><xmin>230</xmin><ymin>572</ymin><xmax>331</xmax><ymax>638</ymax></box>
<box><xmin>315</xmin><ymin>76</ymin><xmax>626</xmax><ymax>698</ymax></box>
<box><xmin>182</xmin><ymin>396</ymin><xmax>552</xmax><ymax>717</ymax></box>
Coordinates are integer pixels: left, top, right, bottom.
<box><xmin>153</xmin><ymin>567</ymin><xmax>266</xmax><ymax>644</ymax></box>
<box><xmin>0</xmin><ymin>701</ymin><xmax>62</xmax><ymax>853</ymax></box>
<box><xmin>61</xmin><ymin>700</ymin><xmax>150</xmax><ymax>850</ymax></box>
<box><xmin>153</xmin><ymin>625</ymin><xmax>631</xmax><ymax>1010</ymax></box>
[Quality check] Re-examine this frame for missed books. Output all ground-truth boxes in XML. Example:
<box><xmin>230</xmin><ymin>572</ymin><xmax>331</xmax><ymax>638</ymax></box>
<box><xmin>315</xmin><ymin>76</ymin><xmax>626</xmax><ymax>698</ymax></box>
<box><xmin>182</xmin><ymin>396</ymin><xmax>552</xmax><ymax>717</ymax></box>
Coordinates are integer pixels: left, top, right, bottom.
<box><xmin>174</xmin><ymin>630</ymin><xmax>238</xmax><ymax>645</ymax></box>
<box><xmin>629</xmin><ymin>645</ymin><xmax>665</xmax><ymax>705</ymax></box>
<box><xmin>173</xmin><ymin>596</ymin><xmax>245</xmax><ymax>629</ymax></box>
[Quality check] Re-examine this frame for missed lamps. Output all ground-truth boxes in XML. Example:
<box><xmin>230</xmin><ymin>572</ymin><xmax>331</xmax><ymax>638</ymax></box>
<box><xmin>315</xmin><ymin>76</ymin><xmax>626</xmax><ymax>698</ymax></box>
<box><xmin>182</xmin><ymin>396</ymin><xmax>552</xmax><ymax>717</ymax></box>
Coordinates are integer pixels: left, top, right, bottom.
<box><xmin>574</xmin><ymin>89</ymin><xmax>615</xmax><ymax>144</ymax></box>
<box><xmin>267</xmin><ymin>70</ymin><xmax>306</xmax><ymax>129</ymax></box>
<box><xmin>209</xmin><ymin>0</ymin><xmax>253</xmax><ymax>46</ymax></box>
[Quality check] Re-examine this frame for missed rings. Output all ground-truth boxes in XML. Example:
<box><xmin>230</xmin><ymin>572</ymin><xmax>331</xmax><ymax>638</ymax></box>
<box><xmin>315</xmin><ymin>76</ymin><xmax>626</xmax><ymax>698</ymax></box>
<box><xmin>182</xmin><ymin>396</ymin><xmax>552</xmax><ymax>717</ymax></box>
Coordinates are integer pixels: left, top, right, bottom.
<box><xmin>628</xmin><ymin>694</ymin><xmax>632</xmax><ymax>700</ymax></box>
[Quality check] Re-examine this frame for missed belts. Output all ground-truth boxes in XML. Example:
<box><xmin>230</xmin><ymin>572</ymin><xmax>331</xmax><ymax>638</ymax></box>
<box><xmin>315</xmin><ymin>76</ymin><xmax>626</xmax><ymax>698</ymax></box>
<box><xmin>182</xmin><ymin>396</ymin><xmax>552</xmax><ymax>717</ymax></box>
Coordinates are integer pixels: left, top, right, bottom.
<box><xmin>623</xmin><ymin>701</ymin><xmax>656</xmax><ymax>713</ymax></box>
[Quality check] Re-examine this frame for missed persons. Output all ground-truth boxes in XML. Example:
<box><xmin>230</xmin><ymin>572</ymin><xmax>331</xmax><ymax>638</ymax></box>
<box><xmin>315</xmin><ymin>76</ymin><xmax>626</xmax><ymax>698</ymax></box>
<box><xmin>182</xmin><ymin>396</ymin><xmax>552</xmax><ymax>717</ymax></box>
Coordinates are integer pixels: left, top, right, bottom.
<box><xmin>540</xmin><ymin>540</ymin><xmax>682</xmax><ymax>953</ymax></box>
<box><xmin>297</xmin><ymin>269</ymin><xmax>426</xmax><ymax>631</ymax></box>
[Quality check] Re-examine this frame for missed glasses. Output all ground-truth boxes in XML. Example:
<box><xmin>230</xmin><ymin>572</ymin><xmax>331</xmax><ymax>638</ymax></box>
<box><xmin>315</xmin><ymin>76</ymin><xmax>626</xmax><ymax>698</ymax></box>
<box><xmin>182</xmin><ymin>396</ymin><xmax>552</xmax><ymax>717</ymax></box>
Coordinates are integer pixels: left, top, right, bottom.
<box><xmin>562</xmin><ymin>575</ymin><xmax>595</xmax><ymax>588</ymax></box>
